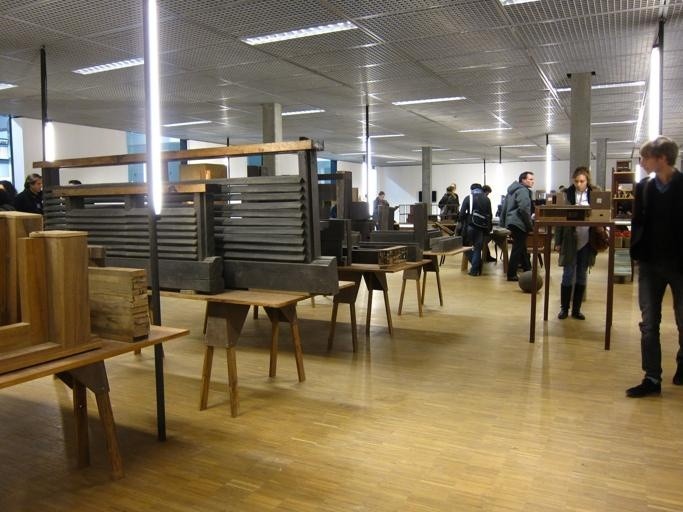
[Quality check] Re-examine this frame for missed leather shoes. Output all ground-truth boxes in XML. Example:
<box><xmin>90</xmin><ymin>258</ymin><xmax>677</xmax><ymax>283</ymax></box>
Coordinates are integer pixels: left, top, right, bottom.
<box><xmin>672</xmin><ymin>351</ymin><xmax>681</xmax><ymax>386</ymax></box>
<box><xmin>558</xmin><ymin>308</ymin><xmax>568</xmax><ymax>319</ymax></box>
<box><xmin>626</xmin><ymin>376</ymin><xmax>661</xmax><ymax>397</ymax></box>
<box><xmin>571</xmin><ymin>310</ymin><xmax>585</xmax><ymax>320</ymax></box>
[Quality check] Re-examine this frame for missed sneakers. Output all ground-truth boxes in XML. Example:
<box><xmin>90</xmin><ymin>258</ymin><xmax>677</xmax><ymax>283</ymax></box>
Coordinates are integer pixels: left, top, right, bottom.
<box><xmin>507</xmin><ymin>274</ymin><xmax>519</xmax><ymax>282</ymax></box>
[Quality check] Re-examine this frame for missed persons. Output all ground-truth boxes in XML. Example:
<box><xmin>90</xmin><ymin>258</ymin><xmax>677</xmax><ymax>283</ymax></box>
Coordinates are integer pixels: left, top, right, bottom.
<box><xmin>623</xmin><ymin>134</ymin><xmax>683</xmax><ymax>398</ymax></box>
<box><xmin>437</xmin><ymin>181</ymin><xmax>505</xmax><ymax>277</ymax></box>
<box><xmin>62</xmin><ymin>179</ymin><xmax>96</xmax><ymax>205</ymax></box>
<box><xmin>499</xmin><ymin>171</ymin><xmax>535</xmax><ymax>282</ymax></box>
<box><xmin>0</xmin><ymin>173</ymin><xmax>43</xmax><ymax>214</ymax></box>
<box><xmin>371</xmin><ymin>191</ymin><xmax>390</xmax><ymax>231</ymax></box>
<box><xmin>553</xmin><ymin>166</ymin><xmax>609</xmax><ymax>322</ymax></box>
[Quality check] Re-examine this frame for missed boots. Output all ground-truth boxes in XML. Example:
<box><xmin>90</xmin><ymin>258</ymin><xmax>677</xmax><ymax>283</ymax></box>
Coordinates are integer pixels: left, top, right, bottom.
<box><xmin>467</xmin><ymin>265</ymin><xmax>483</xmax><ymax>276</ymax></box>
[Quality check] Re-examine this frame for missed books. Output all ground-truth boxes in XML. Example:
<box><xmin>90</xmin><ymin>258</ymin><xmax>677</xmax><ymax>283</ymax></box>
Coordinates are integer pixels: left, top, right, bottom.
<box><xmin>614</xmin><ymin>225</ymin><xmax>632</xmax><ymax>276</ymax></box>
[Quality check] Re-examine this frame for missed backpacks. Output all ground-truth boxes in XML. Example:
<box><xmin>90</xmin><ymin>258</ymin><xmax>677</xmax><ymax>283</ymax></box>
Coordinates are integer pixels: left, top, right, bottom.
<box><xmin>589</xmin><ymin>226</ymin><xmax>609</xmax><ymax>252</ymax></box>
<box><xmin>469</xmin><ymin>209</ymin><xmax>492</xmax><ymax>230</ymax></box>
